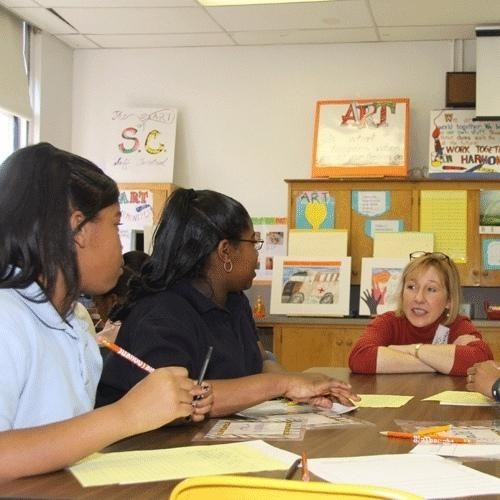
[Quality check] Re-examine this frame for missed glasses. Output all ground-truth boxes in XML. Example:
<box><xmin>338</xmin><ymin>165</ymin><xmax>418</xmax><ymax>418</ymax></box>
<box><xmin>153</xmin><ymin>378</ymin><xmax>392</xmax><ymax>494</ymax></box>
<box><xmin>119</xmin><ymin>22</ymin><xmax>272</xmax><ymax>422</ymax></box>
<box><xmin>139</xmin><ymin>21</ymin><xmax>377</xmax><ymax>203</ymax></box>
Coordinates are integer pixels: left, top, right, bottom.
<box><xmin>217</xmin><ymin>238</ymin><xmax>264</xmax><ymax>250</ymax></box>
<box><xmin>410</xmin><ymin>251</ymin><xmax>450</xmax><ymax>264</ymax></box>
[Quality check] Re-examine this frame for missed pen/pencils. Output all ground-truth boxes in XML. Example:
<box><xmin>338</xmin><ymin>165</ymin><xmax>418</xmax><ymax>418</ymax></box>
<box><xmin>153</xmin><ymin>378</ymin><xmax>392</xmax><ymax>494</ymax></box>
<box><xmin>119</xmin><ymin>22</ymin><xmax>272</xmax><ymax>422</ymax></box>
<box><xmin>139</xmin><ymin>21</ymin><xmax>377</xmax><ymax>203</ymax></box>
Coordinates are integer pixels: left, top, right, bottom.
<box><xmin>416</xmin><ymin>425</ymin><xmax>449</xmax><ymax>434</ymax></box>
<box><xmin>302</xmin><ymin>453</ymin><xmax>308</xmax><ymax>479</ymax></box>
<box><xmin>94</xmin><ymin>318</ymin><xmax>102</xmax><ymax>327</ymax></box>
<box><xmin>376</xmin><ymin>430</ymin><xmax>477</xmax><ymax>443</ymax></box>
<box><xmin>97</xmin><ymin>334</ymin><xmax>155</xmax><ymax>374</ymax></box>
<box><xmin>184</xmin><ymin>346</ymin><xmax>214</xmax><ymax>423</ymax></box>
<box><xmin>288</xmin><ymin>458</ymin><xmax>301</xmax><ymax>473</ymax></box>
<box><xmin>286</xmin><ymin>466</ymin><xmax>299</xmax><ymax>480</ymax></box>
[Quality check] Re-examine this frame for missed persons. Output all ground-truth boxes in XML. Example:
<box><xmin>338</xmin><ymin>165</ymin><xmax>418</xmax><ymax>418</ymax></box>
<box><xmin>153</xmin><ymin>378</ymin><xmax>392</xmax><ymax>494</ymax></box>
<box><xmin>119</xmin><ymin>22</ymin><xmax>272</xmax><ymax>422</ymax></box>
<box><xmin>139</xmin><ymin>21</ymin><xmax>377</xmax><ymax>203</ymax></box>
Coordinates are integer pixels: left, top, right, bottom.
<box><xmin>93</xmin><ymin>251</ymin><xmax>152</xmax><ymax>347</ymax></box>
<box><xmin>465</xmin><ymin>359</ymin><xmax>500</xmax><ymax>402</ymax></box>
<box><xmin>348</xmin><ymin>252</ymin><xmax>494</xmax><ymax>376</ymax></box>
<box><xmin>0</xmin><ymin>141</ymin><xmax>214</xmax><ymax>485</ymax></box>
<box><xmin>94</xmin><ymin>188</ymin><xmax>362</xmax><ymax>427</ymax></box>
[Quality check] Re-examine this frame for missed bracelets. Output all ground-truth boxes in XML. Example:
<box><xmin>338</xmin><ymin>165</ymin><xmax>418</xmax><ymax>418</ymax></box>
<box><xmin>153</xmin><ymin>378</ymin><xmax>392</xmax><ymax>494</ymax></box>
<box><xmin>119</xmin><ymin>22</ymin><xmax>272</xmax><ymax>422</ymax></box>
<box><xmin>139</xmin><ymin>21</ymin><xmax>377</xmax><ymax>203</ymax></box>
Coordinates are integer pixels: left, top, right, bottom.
<box><xmin>415</xmin><ymin>343</ymin><xmax>423</xmax><ymax>359</ymax></box>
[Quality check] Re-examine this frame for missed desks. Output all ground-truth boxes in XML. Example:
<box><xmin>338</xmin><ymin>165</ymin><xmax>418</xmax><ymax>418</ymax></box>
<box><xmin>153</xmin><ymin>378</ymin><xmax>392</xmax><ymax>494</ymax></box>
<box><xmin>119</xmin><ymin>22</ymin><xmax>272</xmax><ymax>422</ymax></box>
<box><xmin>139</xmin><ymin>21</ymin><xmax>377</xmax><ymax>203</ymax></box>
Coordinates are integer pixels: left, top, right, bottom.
<box><xmin>0</xmin><ymin>366</ymin><xmax>500</xmax><ymax>500</ymax></box>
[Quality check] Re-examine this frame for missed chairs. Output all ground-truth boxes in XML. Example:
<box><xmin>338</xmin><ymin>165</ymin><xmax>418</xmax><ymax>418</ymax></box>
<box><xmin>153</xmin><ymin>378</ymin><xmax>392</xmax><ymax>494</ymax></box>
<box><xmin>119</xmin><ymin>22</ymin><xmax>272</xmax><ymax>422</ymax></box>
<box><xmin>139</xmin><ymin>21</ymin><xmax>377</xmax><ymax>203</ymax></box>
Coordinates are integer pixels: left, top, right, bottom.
<box><xmin>169</xmin><ymin>476</ymin><xmax>427</xmax><ymax>500</ymax></box>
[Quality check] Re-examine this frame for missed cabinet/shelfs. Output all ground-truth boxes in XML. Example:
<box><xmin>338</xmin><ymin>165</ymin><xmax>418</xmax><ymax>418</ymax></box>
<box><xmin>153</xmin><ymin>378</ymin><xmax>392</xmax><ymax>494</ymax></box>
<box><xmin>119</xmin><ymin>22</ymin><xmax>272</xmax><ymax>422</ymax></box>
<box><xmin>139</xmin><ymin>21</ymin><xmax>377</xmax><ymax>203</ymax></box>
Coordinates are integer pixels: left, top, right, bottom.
<box><xmin>273</xmin><ymin>324</ymin><xmax>367</xmax><ymax>373</ymax></box>
<box><xmin>476</xmin><ymin>326</ymin><xmax>500</xmax><ymax>365</ymax></box>
<box><xmin>417</xmin><ymin>179</ymin><xmax>499</xmax><ymax>287</ymax></box>
<box><xmin>284</xmin><ymin>175</ymin><xmax>415</xmax><ymax>286</ymax></box>
<box><xmin>115</xmin><ymin>182</ymin><xmax>181</xmax><ymax>224</ymax></box>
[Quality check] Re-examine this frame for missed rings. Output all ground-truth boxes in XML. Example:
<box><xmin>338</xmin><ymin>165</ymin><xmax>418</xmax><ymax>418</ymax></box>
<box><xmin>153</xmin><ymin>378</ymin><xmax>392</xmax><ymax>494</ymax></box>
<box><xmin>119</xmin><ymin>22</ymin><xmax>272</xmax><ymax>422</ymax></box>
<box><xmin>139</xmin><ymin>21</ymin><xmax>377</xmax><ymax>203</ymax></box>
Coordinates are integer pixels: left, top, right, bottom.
<box><xmin>469</xmin><ymin>375</ymin><xmax>473</xmax><ymax>383</ymax></box>
